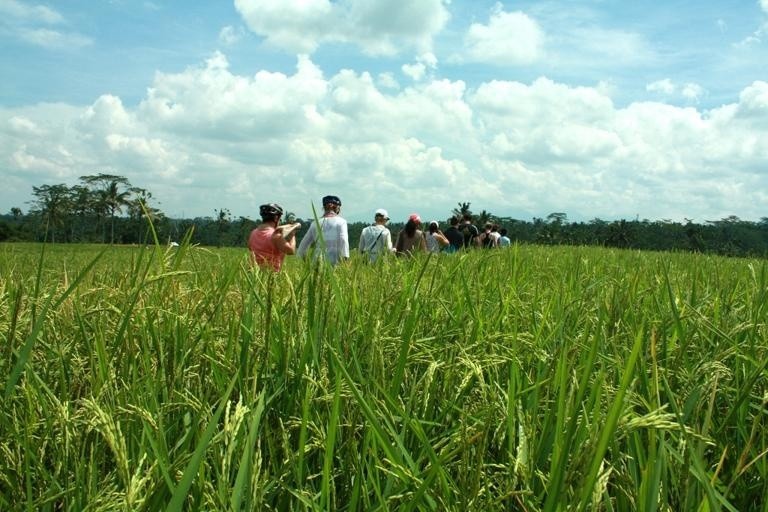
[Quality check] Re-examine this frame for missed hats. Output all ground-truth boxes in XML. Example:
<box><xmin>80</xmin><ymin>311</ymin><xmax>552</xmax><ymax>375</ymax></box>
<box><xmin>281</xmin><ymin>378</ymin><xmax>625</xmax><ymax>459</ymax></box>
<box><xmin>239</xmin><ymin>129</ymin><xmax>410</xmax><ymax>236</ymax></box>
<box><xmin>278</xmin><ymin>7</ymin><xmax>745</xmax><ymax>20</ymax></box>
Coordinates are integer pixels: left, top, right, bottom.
<box><xmin>431</xmin><ymin>220</ymin><xmax>439</xmax><ymax>228</ymax></box>
<box><xmin>375</xmin><ymin>209</ymin><xmax>390</xmax><ymax>220</ymax></box>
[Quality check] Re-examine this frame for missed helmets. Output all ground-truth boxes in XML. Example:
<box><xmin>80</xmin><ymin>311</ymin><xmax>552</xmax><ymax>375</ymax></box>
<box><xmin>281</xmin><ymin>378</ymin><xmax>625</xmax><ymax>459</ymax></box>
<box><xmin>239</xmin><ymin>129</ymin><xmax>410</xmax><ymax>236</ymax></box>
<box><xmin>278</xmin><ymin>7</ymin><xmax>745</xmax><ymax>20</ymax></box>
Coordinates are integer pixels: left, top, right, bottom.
<box><xmin>409</xmin><ymin>214</ymin><xmax>421</xmax><ymax>224</ymax></box>
<box><xmin>323</xmin><ymin>196</ymin><xmax>341</xmax><ymax>207</ymax></box>
<box><xmin>259</xmin><ymin>203</ymin><xmax>284</xmax><ymax>216</ymax></box>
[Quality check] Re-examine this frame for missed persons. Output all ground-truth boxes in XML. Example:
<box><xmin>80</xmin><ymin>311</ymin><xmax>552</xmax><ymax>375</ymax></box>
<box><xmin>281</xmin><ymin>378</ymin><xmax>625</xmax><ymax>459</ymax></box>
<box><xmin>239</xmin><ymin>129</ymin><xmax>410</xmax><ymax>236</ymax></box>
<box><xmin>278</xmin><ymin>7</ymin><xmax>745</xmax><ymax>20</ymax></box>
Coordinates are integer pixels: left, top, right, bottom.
<box><xmin>249</xmin><ymin>203</ymin><xmax>296</xmax><ymax>272</ymax></box>
<box><xmin>297</xmin><ymin>195</ymin><xmax>349</xmax><ymax>272</ymax></box>
<box><xmin>359</xmin><ymin>209</ymin><xmax>397</xmax><ymax>265</ymax></box>
<box><xmin>425</xmin><ymin>215</ymin><xmax>511</xmax><ymax>254</ymax></box>
<box><xmin>395</xmin><ymin>214</ymin><xmax>427</xmax><ymax>258</ymax></box>
